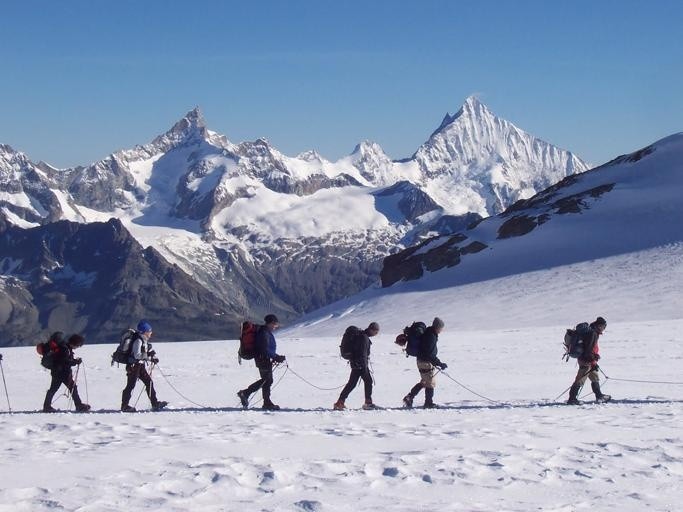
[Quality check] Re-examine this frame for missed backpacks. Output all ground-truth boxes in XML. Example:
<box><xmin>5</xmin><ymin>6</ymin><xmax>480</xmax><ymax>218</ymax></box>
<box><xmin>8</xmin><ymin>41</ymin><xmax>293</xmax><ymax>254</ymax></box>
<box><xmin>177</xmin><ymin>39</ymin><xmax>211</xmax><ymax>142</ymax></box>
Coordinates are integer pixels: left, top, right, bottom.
<box><xmin>563</xmin><ymin>323</ymin><xmax>590</xmax><ymax>357</ymax></box>
<box><xmin>340</xmin><ymin>325</ymin><xmax>360</xmax><ymax>359</ymax></box>
<box><xmin>405</xmin><ymin>321</ymin><xmax>426</xmax><ymax>357</ymax></box>
<box><xmin>42</xmin><ymin>331</ymin><xmax>63</xmax><ymax>370</ymax></box>
<box><xmin>112</xmin><ymin>328</ymin><xmax>143</xmax><ymax>366</ymax></box>
<box><xmin>239</xmin><ymin>320</ymin><xmax>259</xmax><ymax>359</ymax></box>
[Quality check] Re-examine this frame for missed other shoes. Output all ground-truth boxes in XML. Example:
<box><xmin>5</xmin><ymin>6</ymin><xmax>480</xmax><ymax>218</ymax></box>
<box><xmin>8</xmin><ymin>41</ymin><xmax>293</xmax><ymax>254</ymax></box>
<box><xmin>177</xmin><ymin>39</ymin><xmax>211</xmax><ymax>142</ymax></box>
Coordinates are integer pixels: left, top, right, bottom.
<box><xmin>364</xmin><ymin>401</ymin><xmax>376</xmax><ymax>409</ymax></box>
<box><xmin>404</xmin><ymin>396</ymin><xmax>413</xmax><ymax>409</ymax></box>
<box><xmin>262</xmin><ymin>401</ymin><xmax>280</xmax><ymax>410</ymax></box>
<box><xmin>424</xmin><ymin>402</ymin><xmax>439</xmax><ymax>409</ymax></box>
<box><xmin>238</xmin><ymin>391</ymin><xmax>249</xmax><ymax>406</ymax></box>
<box><xmin>76</xmin><ymin>404</ymin><xmax>90</xmax><ymax>410</ymax></box>
<box><xmin>335</xmin><ymin>399</ymin><xmax>346</xmax><ymax>408</ymax></box>
<box><xmin>121</xmin><ymin>404</ymin><xmax>136</xmax><ymax>411</ymax></box>
<box><xmin>45</xmin><ymin>405</ymin><xmax>56</xmax><ymax>412</ymax></box>
<box><xmin>152</xmin><ymin>401</ymin><xmax>166</xmax><ymax>409</ymax></box>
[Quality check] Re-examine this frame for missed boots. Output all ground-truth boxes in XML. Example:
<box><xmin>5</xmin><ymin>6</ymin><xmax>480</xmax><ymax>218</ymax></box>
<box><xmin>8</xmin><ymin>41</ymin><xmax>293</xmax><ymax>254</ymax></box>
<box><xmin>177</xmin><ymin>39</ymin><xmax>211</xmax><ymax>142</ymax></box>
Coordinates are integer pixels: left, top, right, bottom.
<box><xmin>591</xmin><ymin>382</ymin><xmax>611</xmax><ymax>403</ymax></box>
<box><xmin>567</xmin><ymin>383</ymin><xmax>582</xmax><ymax>404</ymax></box>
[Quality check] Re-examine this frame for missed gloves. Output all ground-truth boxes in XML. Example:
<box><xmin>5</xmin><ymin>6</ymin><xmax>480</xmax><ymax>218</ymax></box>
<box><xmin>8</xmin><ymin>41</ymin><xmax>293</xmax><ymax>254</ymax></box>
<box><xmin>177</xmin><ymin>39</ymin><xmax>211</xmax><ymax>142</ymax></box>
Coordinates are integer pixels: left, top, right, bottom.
<box><xmin>440</xmin><ymin>364</ymin><xmax>447</xmax><ymax>370</ymax></box>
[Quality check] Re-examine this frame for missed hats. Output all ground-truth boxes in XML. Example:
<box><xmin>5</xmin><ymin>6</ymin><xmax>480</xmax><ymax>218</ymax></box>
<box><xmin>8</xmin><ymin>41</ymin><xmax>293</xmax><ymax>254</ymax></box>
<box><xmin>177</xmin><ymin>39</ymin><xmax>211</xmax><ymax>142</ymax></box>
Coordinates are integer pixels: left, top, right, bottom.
<box><xmin>137</xmin><ymin>322</ymin><xmax>152</xmax><ymax>332</ymax></box>
<box><xmin>264</xmin><ymin>314</ymin><xmax>277</xmax><ymax>322</ymax></box>
<box><xmin>596</xmin><ymin>318</ymin><xmax>606</xmax><ymax>325</ymax></box>
<box><xmin>368</xmin><ymin>322</ymin><xmax>380</xmax><ymax>331</ymax></box>
<box><xmin>433</xmin><ymin>318</ymin><xmax>444</xmax><ymax>329</ymax></box>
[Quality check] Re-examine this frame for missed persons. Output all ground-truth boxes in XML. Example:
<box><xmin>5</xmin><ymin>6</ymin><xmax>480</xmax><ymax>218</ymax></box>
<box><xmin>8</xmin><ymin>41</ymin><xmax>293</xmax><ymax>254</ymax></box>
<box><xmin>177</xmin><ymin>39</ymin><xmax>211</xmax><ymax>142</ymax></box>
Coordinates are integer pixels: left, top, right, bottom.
<box><xmin>403</xmin><ymin>316</ymin><xmax>448</xmax><ymax>407</ymax></box>
<box><xmin>334</xmin><ymin>321</ymin><xmax>380</xmax><ymax>410</ymax></box>
<box><xmin>120</xmin><ymin>319</ymin><xmax>167</xmax><ymax>412</ymax></box>
<box><xmin>42</xmin><ymin>334</ymin><xmax>90</xmax><ymax>412</ymax></box>
<box><xmin>236</xmin><ymin>313</ymin><xmax>285</xmax><ymax>410</ymax></box>
<box><xmin>567</xmin><ymin>316</ymin><xmax>612</xmax><ymax>405</ymax></box>
